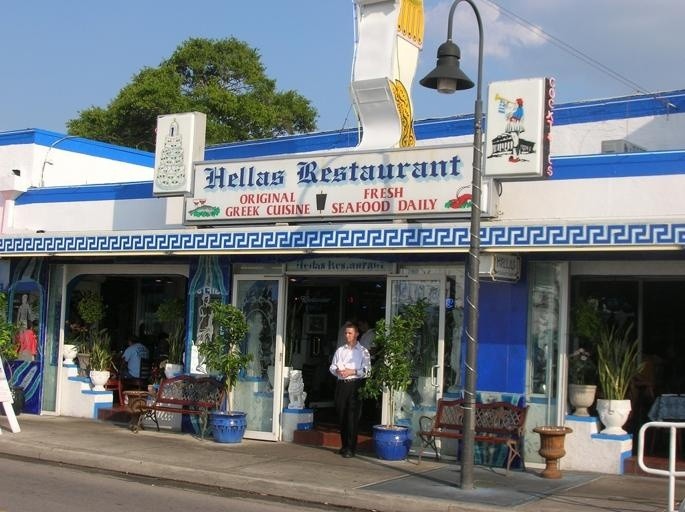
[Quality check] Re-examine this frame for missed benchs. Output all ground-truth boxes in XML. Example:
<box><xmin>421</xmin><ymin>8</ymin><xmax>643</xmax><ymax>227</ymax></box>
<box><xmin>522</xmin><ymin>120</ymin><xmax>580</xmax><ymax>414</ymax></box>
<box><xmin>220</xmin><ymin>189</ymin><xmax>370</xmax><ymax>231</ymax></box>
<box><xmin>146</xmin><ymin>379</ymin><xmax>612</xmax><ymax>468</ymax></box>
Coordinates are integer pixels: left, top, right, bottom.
<box><xmin>129</xmin><ymin>374</ymin><xmax>228</xmax><ymax>441</ymax></box>
<box><xmin>417</xmin><ymin>397</ymin><xmax>530</xmax><ymax>480</ymax></box>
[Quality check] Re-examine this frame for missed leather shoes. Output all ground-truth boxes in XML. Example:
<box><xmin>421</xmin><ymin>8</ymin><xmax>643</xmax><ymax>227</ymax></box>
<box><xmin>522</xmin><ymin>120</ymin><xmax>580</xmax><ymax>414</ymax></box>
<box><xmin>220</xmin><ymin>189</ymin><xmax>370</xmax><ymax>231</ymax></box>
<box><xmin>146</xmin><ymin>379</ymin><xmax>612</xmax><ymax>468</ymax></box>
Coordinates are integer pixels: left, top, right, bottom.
<box><xmin>344</xmin><ymin>450</ymin><xmax>355</xmax><ymax>457</ymax></box>
<box><xmin>340</xmin><ymin>447</ymin><xmax>347</xmax><ymax>453</ymax></box>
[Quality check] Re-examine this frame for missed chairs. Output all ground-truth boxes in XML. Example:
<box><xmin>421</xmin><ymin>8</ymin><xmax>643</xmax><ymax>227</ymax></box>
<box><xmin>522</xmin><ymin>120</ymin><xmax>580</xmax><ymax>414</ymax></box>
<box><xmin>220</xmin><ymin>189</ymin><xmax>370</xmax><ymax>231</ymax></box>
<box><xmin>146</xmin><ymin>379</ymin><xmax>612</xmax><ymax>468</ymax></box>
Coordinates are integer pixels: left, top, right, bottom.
<box><xmin>99</xmin><ymin>343</ymin><xmax>153</xmax><ymax>422</ymax></box>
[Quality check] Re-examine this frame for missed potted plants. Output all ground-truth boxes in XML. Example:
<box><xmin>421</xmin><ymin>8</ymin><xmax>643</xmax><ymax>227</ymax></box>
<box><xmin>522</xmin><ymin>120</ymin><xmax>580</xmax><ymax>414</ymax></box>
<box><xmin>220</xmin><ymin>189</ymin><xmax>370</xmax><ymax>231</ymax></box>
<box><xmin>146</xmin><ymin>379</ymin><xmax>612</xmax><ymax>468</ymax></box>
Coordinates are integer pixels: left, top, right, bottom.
<box><xmin>567</xmin><ymin>298</ymin><xmax>607</xmax><ymax>417</ymax></box>
<box><xmin>593</xmin><ymin>315</ymin><xmax>646</xmax><ymax>436</ymax></box>
<box><xmin>198</xmin><ymin>298</ymin><xmax>247</xmax><ymax>443</ymax></box>
<box><xmin>76</xmin><ymin>291</ymin><xmax>106</xmax><ymax>377</ymax></box>
<box><xmin>358</xmin><ymin>296</ymin><xmax>432</xmax><ymax>462</ymax></box>
<box><xmin>1</xmin><ymin>292</ymin><xmax>25</xmax><ymax>416</ymax></box>
<box><xmin>154</xmin><ymin>294</ymin><xmax>182</xmax><ymax>377</ymax></box>
<box><xmin>64</xmin><ymin>324</ymin><xmax>85</xmax><ymax>365</ymax></box>
<box><xmin>89</xmin><ymin>333</ymin><xmax>110</xmax><ymax>392</ymax></box>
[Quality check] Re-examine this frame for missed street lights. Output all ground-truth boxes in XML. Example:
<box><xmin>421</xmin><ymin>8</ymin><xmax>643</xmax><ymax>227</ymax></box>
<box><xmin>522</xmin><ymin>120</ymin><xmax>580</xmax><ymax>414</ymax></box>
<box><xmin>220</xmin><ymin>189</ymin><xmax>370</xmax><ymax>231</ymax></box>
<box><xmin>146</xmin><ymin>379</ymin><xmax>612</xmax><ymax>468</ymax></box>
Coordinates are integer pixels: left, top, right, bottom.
<box><xmin>422</xmin><ymin>0</ymin><xmax>485</xmax><ymax>496</ymax></box>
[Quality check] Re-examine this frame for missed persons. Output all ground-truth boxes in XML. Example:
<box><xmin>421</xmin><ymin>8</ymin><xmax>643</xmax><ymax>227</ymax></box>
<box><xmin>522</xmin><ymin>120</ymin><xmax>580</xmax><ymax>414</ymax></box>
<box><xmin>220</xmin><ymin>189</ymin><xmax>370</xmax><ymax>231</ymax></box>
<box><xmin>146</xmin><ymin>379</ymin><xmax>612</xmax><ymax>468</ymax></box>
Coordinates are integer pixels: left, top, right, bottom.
<box><xmin>328</xmin><ymin>315</ymin><xmax>379</xmax><ymax>364</ymax></box>
<box><xmin>504</xmin><ymin>98</ymin><xmax>524</xmax><ymax>163</ymax></box>
<box><xmin>329</xmin><ymin>324</ymin><xmax>373</xmax><ymax>460</ymax></box>
<box><xmin>14</xmin><ymin>322</ymin><xmax>37</xmax><ymax>361</ymax></box>
<box><xmin>120</xmin><ymin>335</ymin><xmax>150</xmax><ymax>379</ymax></box>
